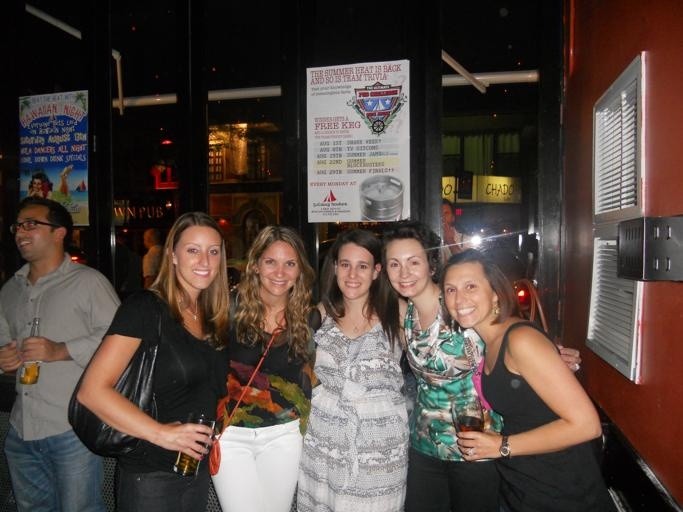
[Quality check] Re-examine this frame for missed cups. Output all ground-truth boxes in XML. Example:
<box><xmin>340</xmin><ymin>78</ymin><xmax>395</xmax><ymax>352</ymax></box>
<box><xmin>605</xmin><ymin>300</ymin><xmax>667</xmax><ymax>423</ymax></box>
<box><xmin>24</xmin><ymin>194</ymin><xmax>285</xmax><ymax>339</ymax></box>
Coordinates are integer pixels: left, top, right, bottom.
<box><xmin>172</xmin><ymin>411</ymin><xmax>216</xmax><ymax>479</ymax></box>
<box><xmin>450</xmin><ymin>393</ymin><xmax>487</xmax><ymax>439</ymax></box>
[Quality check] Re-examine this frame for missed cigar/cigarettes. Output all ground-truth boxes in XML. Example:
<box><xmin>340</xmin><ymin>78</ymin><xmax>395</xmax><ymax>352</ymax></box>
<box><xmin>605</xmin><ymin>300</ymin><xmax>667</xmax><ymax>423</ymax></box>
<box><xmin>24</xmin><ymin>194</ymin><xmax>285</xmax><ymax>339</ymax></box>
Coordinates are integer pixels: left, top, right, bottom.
<box><xmin>442</xmin><ymin>222</ymin><xmax>445</xmax><ymax>225</ymax></box>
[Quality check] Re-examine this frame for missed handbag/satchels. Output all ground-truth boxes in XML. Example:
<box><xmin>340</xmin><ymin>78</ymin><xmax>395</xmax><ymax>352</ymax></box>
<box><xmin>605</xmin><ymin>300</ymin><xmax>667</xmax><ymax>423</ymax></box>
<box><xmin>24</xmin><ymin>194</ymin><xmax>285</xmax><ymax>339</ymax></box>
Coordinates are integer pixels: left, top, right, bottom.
<box><xmin>67</xmin><ymin>288</ymin><xmax>164</xmax><ymax>459</ymax></box>
<box><xmin>209</xmin><ymin>437</ymin><xmax>221</xmax><ymax>476</ymax></box>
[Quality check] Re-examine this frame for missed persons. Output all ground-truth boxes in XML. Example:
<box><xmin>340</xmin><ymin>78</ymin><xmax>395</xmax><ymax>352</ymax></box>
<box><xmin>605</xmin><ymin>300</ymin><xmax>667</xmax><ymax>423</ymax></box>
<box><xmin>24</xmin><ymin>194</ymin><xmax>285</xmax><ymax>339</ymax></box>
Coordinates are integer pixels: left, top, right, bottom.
<box><xmin>76</xmin><ymin>211</ymin><xmax>228</xmax><ymax>512</ymax></box>
<box><xmin>209</xmin><ymin>224</ymin><xmax>321</xmax><ymax>512</ymax></box>
<box><xmin>143</xmin><ymin>227</ymin><xmax>162</xmax><ymax>289</ymax></box>
<box><xmin>296</xmin><ymin>228</ymin><xmax>410</xmax><ymax>512</ymax></box>
<box><xmin>440</xmin><ymin>195</ymin><xmax>479</xmax><ymax>273</ymax></box>
<box><xmin>438</xmin><ymin>247</ymin><xmax>618</xmax><ymax>512</ymax></box>
<box><xmin>0</xmin><ymin>194</ymin><xmax>122</xmax><ymax>512</ymax></box>
<box><xmin>382</xmin><ymin>218</ymin><xmax>582</xmax><ymax>512</ymax></box>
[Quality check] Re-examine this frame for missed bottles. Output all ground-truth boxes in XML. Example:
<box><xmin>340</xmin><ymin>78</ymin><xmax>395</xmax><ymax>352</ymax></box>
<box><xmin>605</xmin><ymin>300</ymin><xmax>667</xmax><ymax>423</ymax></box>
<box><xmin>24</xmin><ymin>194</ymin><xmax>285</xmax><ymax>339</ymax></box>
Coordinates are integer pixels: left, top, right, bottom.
<box><xmin>20</xmin><ymin>314</ymin><xmax>43</xmax><ymax>386</ymax></box>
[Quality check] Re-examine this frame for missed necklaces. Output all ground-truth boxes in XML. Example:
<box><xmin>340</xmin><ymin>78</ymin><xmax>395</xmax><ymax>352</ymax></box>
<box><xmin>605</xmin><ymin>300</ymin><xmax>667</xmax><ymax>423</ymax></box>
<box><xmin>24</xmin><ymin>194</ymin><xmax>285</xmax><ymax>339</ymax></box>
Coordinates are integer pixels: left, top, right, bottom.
<box><xmin>183</xmin><ymin>300</ymin><xmax>199</xmax><ymax>320</ymax></box>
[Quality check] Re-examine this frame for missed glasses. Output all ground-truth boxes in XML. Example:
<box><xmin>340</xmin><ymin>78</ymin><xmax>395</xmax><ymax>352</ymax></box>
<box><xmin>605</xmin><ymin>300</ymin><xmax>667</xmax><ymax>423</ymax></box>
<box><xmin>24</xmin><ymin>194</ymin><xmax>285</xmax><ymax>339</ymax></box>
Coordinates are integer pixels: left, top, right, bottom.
<box><xmin>9</xmin><ymin>219</ymin><xmax>60</xmax><ymax>234</ymax></box>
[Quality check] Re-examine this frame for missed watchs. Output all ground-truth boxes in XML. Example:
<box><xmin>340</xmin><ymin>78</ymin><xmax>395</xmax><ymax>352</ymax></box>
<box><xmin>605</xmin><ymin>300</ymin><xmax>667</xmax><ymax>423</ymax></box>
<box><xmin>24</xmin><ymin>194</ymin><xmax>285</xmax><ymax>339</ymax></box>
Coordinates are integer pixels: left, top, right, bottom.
<box><xmin>499</xmin><ymin>435</ymin><xmax>510</xmax><ymax>460</ymax></box>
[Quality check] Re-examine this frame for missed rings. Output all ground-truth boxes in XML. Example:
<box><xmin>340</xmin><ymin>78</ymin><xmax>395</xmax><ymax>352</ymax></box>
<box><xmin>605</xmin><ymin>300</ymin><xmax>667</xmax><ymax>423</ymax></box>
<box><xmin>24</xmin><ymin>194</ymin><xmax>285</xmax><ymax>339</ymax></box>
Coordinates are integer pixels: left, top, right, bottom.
<box><xmin>467</xmin><ymin>448</ymin><xmax>472</xmax><ymax>455</ymax></box>
<box><xmin>575</xmin><ymin>363</ymin><xmax>579</xmax><ymax>371</ymax></box>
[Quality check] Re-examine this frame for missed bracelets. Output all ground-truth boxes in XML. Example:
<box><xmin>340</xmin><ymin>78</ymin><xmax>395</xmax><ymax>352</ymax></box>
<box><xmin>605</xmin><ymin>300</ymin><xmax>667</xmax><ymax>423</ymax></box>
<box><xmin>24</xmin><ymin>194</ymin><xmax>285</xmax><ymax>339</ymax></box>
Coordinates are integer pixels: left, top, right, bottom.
<box><xmin>445</xmin><ymin>238</ymin><xmax>457</xmax><ymax>245</ymax></box>
